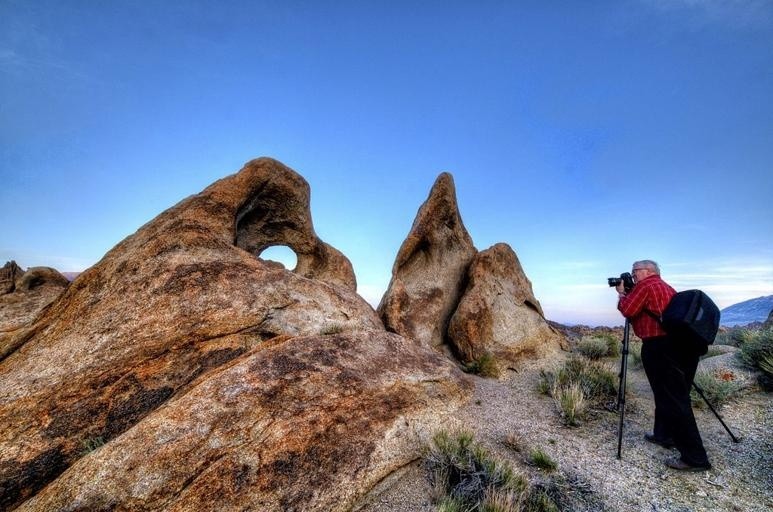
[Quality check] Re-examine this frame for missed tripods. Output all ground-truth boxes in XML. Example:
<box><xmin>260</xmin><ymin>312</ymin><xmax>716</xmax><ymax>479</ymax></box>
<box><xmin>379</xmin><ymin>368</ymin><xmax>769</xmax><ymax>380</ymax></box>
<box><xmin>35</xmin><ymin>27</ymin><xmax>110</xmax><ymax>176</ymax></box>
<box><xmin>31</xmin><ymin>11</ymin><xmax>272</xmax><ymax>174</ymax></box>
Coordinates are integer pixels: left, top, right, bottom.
<box><xmin>617</xmin><ymin>316</ymin><xmax>742</xmax><ymax>459</ymax></box>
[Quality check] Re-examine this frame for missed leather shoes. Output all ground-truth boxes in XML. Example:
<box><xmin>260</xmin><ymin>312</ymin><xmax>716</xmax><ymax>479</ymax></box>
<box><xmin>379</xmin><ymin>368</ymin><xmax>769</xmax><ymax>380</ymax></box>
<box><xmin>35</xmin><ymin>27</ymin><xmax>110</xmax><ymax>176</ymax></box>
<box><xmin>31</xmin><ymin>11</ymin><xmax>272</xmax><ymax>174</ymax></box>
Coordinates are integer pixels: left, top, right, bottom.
<box><xmin>644</xmin><ymin>431</ymin><xmax>671</xmax><ymax>449</ymax></box>
<box><xmin>666</xmin><ymin>457</ymin><xmax>710</xmax><ymax>472</ymax></box>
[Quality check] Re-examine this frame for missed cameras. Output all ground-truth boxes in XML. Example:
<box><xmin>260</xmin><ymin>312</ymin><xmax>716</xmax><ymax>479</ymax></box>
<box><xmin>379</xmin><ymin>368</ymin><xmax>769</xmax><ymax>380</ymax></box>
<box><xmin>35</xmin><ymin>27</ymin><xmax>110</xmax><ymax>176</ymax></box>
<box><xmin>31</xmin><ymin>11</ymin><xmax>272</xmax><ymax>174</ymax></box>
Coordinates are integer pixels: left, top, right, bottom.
<box><xmin>608</xmin><ymin>272</ymin><xmax>634</xmax><ymax>293</ymax></box>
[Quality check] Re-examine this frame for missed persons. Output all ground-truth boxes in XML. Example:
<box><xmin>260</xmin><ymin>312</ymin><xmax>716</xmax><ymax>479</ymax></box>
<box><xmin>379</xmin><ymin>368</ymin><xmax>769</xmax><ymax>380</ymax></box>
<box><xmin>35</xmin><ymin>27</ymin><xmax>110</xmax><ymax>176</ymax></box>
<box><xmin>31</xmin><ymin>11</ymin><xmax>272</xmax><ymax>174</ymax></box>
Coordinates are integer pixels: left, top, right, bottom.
<box><xmin>616</xmin><ymin>259</ymin><xmax>712</xmax><ymax>472</ymax></box>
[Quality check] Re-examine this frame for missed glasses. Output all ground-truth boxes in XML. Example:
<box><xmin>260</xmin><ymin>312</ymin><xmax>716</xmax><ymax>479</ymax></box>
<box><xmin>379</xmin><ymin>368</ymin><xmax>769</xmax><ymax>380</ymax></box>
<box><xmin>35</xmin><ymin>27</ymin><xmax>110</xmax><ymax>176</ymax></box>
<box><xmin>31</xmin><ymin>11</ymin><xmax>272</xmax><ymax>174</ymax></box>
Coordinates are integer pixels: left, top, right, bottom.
<box><xmin>632</xmin><ymin>268</ymin><xmax>647</xmax><ymax>273</ymax></box>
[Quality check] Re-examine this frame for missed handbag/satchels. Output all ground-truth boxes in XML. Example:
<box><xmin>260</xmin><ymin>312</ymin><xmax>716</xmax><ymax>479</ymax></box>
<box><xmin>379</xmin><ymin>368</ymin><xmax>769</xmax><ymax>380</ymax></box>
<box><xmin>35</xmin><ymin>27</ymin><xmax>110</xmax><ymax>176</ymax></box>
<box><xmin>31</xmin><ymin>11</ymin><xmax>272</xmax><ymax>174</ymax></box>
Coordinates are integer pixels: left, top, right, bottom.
<box><xmin>660</xmin><ymin>289</ymin><xmax>720</xmax><ymax>354</ymax></box>
